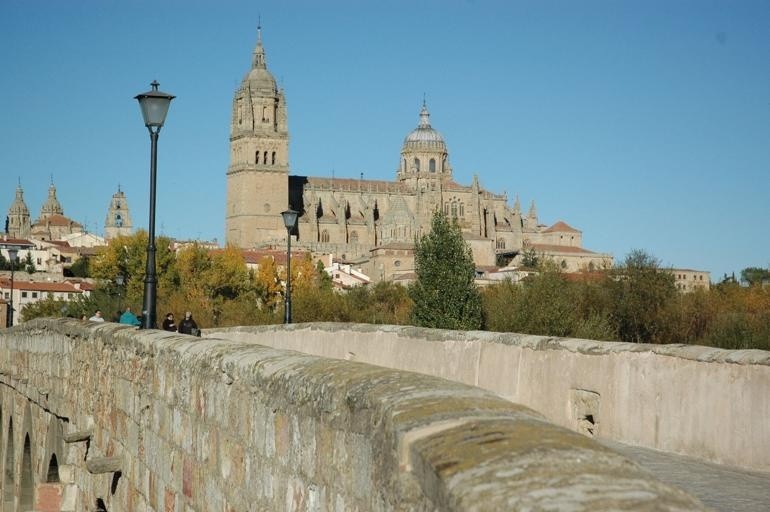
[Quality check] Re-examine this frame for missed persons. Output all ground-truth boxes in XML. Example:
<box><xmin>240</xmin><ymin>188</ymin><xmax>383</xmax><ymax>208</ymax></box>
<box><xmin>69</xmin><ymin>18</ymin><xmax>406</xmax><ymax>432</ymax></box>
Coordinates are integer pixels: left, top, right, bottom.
<box><xmin>66</xmin><ymin>305</ymin><xmax>143</xmax><ymax>328</ymax></box>
<box><xmin>178</xmin><ymin>310</ymin><xmax>198</xmax><ymax>335</ymax></box>
<box><xmin>163</xmin><ymin>313</ymin><xmax>178</xmax><ymax>332</ymax></box>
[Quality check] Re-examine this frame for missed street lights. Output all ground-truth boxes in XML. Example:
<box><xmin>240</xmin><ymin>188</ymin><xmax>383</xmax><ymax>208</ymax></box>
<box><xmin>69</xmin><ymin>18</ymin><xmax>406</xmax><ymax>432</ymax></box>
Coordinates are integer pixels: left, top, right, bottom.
<box><xmin>280</xmin><ymin>204</ymin><xmax>300</xmax><ymax>324</ymax></box>
<box><xmin>132</xmin><ymin>79</ymin><xmax>177</xmax><ymax>331</ymax></box>
<box><xmin>5</xmin><ymin>249</ymin><xmax>18</xmax><ymax>328</ymax></box>
